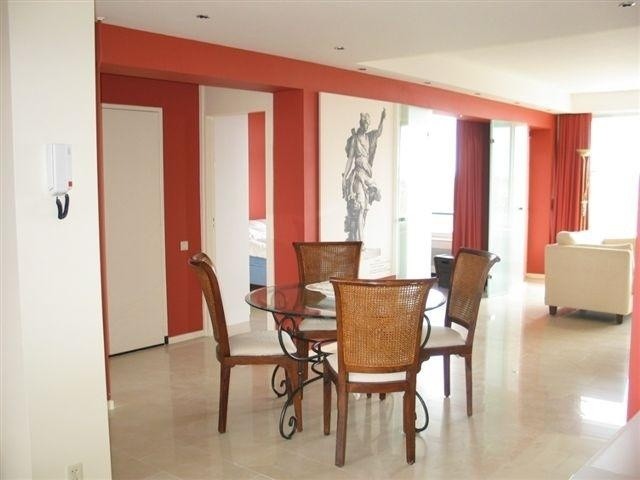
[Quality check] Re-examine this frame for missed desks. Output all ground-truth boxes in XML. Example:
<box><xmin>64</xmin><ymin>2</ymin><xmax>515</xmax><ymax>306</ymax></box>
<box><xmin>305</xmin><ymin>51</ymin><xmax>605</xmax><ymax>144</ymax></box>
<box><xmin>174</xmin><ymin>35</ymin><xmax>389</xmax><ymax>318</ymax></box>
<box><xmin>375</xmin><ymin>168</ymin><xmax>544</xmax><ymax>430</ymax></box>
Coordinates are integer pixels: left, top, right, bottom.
<box><xmin>244</xmin><ymin>278</ymin><xmax>452</xmax><ymax>440</ymax></box>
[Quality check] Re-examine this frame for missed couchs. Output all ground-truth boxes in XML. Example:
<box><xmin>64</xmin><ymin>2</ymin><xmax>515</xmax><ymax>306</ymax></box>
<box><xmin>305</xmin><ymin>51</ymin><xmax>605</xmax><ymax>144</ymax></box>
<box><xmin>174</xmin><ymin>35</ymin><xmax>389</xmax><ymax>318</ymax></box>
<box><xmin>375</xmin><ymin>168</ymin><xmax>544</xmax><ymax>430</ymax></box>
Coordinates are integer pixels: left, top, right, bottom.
<box><xmin>542</xmin><ymin>228</ymin><xmax>636</xmax><ymax>325</ymax></box>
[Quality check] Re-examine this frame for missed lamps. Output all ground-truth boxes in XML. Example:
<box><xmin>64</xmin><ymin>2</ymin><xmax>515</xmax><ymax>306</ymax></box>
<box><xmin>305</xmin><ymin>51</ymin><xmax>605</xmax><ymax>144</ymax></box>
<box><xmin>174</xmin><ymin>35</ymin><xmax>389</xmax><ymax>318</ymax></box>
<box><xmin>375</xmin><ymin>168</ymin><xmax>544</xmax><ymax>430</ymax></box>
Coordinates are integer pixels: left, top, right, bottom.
<box><xmin>575</xmin><ymin>146</ymin><xmax>596</xmax><ymax>232</ymax></box>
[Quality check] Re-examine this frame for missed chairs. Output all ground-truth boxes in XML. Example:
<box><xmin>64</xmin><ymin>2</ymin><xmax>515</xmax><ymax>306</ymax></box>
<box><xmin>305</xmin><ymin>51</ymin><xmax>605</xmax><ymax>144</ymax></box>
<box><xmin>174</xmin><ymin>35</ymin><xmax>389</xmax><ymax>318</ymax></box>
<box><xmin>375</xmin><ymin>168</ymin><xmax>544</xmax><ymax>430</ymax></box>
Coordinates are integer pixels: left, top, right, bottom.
<box><xmin>403</xmin><ymin>248</ymin><xmax>501</xmax><ymax>419</ymax></box>
<box><xmin>292</xmin><ymin>239</ymin><xmax>366</xmax><ymax>392</ymax></box>
<box><xmin>318</xmin><ymin>273</ymin><xmax>439</xmax><ymax>468</ymax></box>
<box><xmin>187</xmin><ymin>251</ymin><xmax>307</xmax><ymax>436</ymax></box>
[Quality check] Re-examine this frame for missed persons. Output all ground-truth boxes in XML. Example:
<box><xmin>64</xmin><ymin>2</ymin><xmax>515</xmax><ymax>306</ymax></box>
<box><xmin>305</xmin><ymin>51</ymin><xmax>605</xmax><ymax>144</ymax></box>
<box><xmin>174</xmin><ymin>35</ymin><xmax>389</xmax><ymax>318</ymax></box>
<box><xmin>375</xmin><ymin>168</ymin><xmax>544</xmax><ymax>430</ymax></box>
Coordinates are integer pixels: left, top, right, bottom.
<box><xmin>339</xmin><ymin>104</ymin><xmax>387</xmax><ymax>255</ymax></box>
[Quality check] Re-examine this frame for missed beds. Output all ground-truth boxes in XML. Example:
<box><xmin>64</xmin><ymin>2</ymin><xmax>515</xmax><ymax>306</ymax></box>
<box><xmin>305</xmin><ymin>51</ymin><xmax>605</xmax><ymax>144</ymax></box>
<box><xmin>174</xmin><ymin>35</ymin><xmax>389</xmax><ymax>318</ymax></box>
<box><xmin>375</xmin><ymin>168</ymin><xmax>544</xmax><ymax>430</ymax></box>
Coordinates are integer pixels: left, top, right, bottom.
<box><xmin>247</xmin><ymin>216</ymin><xmax>267</xmax><ymax>287</ymax></box>
